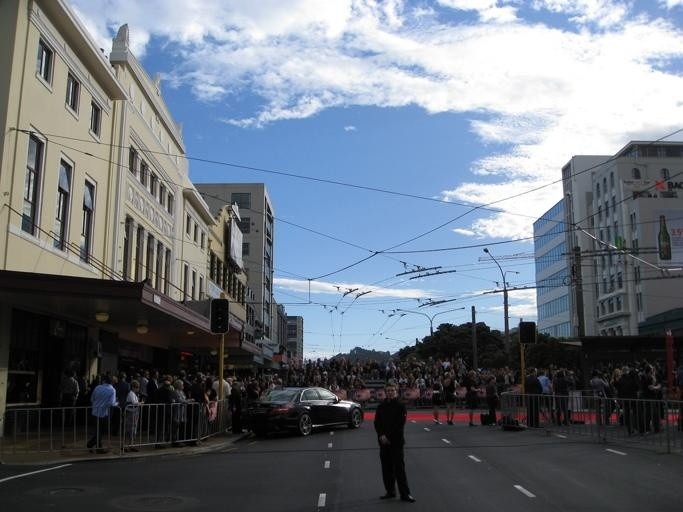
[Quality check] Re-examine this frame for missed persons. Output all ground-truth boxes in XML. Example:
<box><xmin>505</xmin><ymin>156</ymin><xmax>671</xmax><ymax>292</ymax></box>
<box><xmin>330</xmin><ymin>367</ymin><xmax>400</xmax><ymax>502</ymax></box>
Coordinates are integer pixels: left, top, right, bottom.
<box><xmin>57</xmin><ymin>351</ymin><xmax>683</xmax><ymax>454</ymax></box>
<box><xmin>374</xmin><ymin>383</ymin><xmax>416</xmax><ymax>501</ymax></box>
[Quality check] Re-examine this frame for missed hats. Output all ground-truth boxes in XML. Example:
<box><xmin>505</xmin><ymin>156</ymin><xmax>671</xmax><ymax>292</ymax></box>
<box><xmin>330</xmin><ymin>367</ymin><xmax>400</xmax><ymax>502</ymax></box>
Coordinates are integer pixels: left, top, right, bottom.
<box><xmin>240</xmin><ymin>385</ymin><xmax>364</xmax><ymax>440</ymax></box>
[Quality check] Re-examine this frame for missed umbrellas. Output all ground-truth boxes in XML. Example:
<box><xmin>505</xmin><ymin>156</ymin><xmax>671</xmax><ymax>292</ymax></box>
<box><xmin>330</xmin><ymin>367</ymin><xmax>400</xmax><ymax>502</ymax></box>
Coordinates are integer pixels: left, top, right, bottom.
<box><xmin>400</xmin><ymin>495</ymin><xmax>414</xmax><ymax>502</ymax></box>
<box><xmin>379</xmin><ymin>494</ymin><xmax>395</xmax><ymax>499</ymax></box>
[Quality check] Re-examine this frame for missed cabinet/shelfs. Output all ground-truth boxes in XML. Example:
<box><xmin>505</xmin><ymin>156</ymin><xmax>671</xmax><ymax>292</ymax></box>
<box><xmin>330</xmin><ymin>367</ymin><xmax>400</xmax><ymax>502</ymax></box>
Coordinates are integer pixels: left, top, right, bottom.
<box><xmin>657</xmin><ymin>214</ymin><xmax>672</xmax><ymax>261</ymax></box>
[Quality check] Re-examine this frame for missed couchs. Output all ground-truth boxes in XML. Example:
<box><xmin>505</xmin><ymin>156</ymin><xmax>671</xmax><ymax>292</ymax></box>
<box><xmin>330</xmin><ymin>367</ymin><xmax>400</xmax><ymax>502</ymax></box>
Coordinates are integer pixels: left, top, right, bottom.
<box><xmin>209</xmin><ymin>297</ymin><xmax>230</xmax><ymax>337</ymax></box>
<box><xmin>517</xmin><ymin>320</ymin><xmax>538</xmax><ymax>346</ymax></box>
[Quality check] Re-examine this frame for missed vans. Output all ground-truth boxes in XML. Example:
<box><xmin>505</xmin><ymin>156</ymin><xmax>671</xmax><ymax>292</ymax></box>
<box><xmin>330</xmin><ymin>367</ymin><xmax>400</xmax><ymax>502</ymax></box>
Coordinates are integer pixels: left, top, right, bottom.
<box><xmin>433</xmin><ymin>418</ymin><xmax>456</xmax><ymax>427</ymax></box>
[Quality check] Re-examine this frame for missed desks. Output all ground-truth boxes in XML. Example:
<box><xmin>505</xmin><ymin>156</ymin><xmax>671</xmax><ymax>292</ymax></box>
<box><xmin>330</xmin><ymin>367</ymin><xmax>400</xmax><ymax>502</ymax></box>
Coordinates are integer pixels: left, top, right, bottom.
<box><xmin>394</xmin><ymin>305</ymin><xmax>466</xmax><ymax>358</ymax></box>
<box><xmin>476</xmin><ymin>245</ymin><xmax>522</xmax><ymax>368</ymax></box>
